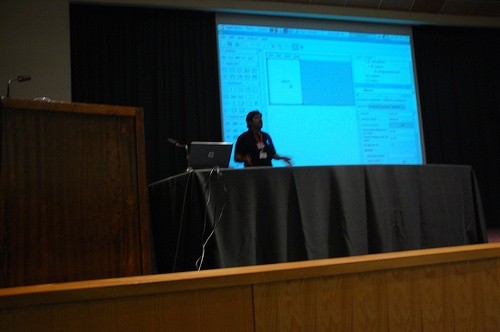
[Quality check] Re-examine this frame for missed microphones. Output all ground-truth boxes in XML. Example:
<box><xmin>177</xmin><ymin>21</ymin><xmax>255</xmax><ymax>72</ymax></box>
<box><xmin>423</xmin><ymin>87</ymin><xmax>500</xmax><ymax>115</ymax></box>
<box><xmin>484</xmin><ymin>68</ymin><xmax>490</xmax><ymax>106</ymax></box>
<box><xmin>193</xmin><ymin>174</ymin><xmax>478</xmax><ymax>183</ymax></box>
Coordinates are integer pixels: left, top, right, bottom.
<box><xmin>7</xmin><ymin>76</ymin><xmax>31</xmax><ymax>98</ymax></box>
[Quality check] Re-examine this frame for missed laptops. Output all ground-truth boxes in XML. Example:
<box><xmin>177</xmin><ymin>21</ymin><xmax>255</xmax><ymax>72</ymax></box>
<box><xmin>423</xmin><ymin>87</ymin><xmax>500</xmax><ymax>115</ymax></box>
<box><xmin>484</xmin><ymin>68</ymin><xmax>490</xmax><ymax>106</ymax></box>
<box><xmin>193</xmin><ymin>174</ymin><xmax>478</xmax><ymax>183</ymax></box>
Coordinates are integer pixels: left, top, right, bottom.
<box><xmin>189</xmin><ymin>142</ymin><xmax>233</xmax><ymax>168</ymax></box>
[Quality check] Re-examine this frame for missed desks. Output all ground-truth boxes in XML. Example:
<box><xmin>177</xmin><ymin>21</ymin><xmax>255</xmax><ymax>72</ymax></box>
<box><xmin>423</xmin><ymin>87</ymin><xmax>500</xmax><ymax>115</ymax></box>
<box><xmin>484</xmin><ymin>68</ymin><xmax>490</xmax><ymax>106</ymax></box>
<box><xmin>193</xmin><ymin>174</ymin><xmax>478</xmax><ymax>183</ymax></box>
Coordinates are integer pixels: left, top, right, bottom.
<box><xmin>146</xmin><ymin>165</ymin><xmax>480</xmax><ymax>272</ymax></box>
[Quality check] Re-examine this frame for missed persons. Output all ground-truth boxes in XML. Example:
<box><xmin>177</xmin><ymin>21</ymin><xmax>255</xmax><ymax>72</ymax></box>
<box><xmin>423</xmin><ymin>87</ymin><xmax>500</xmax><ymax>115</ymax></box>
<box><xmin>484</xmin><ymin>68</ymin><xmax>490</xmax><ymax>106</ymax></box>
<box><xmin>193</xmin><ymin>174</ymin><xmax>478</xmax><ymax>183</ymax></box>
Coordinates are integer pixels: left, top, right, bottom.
<box><xmin>234</xmin><ymin>111</ymin><xmax>292</xmax><ymax>168</ymax></box>
